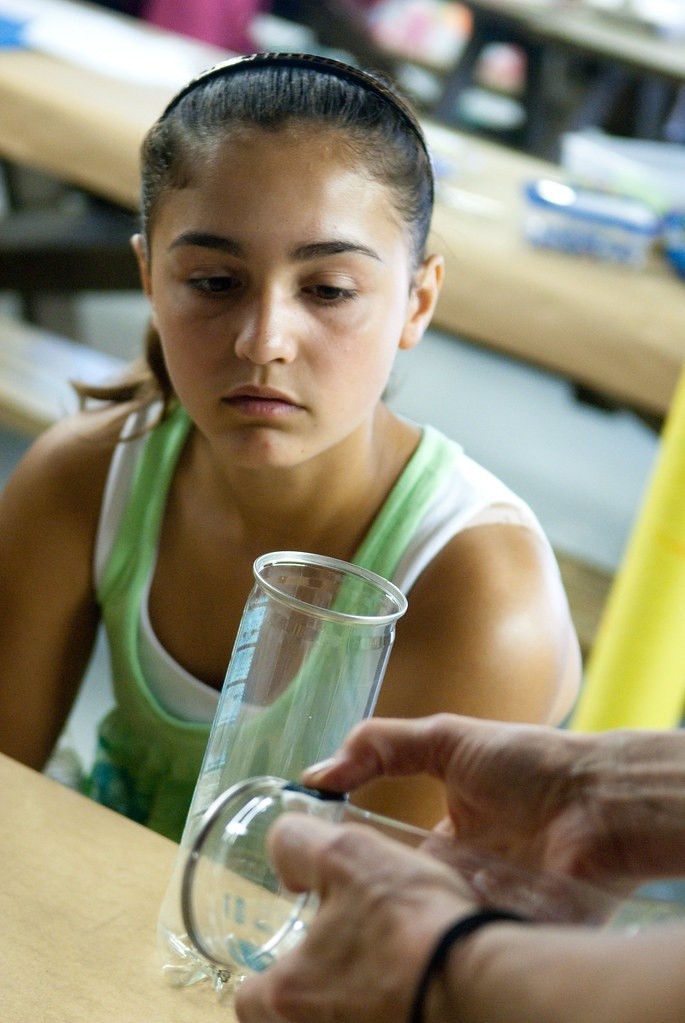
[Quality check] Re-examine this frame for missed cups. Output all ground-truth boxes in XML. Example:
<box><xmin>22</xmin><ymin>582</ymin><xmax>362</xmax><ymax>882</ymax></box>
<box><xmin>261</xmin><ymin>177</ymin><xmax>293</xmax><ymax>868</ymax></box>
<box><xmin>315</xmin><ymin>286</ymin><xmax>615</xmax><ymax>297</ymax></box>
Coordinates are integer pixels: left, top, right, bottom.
<box><xmin>149</xmin><ymin>549</ymin><xmax>411</xmax><ymax>1002</ymax></box>
<box><xmin>181</xmin><ymin>776</ymin><xmax>685</xmax><ymax>989</ymax></box>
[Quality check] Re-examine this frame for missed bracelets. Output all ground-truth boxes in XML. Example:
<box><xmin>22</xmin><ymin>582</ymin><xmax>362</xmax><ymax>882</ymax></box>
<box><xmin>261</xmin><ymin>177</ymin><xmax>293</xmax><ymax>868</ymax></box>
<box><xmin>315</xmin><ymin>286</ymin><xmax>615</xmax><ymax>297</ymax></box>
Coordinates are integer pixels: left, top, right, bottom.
<box><xmin>403</xmin><ymin>906</ymin><xmax>524</xmax><ymax>1023</ymax></box>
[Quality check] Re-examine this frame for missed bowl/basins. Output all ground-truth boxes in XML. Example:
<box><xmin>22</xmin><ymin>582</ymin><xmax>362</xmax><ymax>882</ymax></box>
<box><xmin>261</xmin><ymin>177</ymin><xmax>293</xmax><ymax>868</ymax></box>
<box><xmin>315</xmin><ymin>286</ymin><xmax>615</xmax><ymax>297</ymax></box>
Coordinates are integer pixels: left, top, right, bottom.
<box><xmin>523</xmin><ymin>179</ymin><xmax>664</xmax><ymax>266</ymax></box>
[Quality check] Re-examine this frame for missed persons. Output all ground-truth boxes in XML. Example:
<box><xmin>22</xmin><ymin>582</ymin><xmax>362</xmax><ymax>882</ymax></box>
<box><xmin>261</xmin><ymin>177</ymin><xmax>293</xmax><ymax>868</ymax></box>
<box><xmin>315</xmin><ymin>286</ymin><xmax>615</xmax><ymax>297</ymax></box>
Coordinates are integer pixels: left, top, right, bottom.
<box><xmin>0</xmin><ymin>50</ymin><xmax>583</xmax><ymax>913</ymax></box>
<box><xmin>233</xmin><ymin>710</ymin><xmax>685</xmax><ymax>1023</ymax></box>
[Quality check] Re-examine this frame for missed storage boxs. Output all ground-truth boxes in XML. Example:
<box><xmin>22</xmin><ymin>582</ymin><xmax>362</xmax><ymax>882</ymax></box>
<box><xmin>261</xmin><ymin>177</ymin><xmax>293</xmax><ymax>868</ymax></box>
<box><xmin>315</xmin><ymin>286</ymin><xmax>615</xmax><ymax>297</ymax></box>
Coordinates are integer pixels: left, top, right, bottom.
<box><xmin>530</xmin><ymin>171</ymin><xmax>653</xmax><ymax>267</ymax></box>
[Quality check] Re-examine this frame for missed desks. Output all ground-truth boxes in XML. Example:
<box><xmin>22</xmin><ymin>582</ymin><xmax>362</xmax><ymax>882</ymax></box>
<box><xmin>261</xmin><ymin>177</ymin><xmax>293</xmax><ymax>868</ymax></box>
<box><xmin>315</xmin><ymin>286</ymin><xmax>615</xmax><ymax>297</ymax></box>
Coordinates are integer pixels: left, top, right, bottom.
<box><xmin>0</xmin><ymin>0</ymin><xmax>685</xmax><ymax>419</ymax></box>
<box><xmin>0</xmin><ymin>747</ymin><xmax>324</xmax><ymax>1023</ymax></box>
